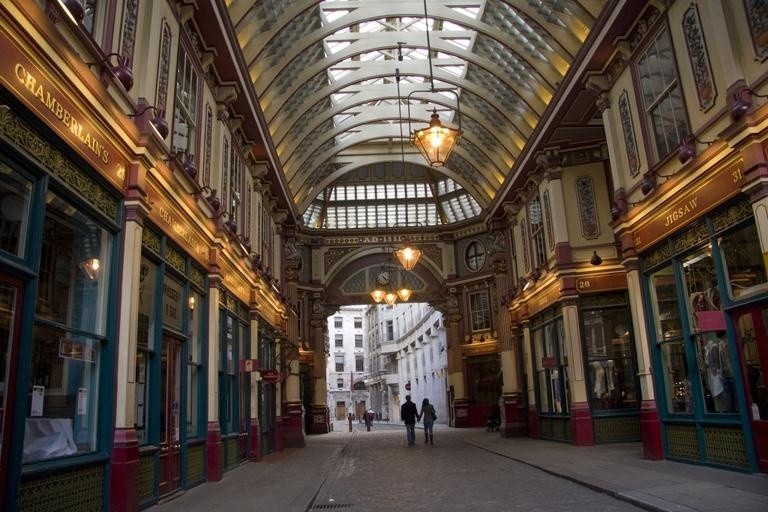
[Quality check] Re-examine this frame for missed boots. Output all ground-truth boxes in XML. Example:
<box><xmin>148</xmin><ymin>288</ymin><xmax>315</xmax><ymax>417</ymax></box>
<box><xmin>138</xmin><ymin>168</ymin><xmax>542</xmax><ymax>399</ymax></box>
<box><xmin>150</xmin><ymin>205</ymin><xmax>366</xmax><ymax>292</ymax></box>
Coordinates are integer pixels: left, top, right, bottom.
<box><xmin>424</xmin><ymin>433</ymin><xmax>428</xmax><ymax>443</ymax></box>
<box><xmin>430</xmin><ymin>435</ymin><xmax>433</xmax><ymax>444</ymax></box>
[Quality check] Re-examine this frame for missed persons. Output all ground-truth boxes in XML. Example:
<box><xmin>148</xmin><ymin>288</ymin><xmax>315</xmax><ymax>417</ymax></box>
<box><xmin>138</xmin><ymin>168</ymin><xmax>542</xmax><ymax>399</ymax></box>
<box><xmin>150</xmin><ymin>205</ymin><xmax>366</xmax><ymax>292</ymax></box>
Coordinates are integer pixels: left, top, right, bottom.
<box><xmin>344</xmin><ymin>406</ymin><xmax>382</xmax><ymax>431</ymax></box>
<box><xmin>589</xmin><ymin>359</ymin><xmax>606</xmax><ymax>400</ymax></box>
<box><xmin>400</xmin><ymin>394</ymin><xmax>417</xmax><ymax>445</ymax></box>
<box><xmin>417</xmin><ymin>398</ymin><xmax>437</xmax><ymax>445</ymax></box>
<box><xmin>603</xmin><ymin>360</ymin><xmax>624</xmax><ymax>408</ymax></box>
<box><xmin>700</xmin><ymin>329</ymin><xmax>733</xmax><ymax>412</ymax></box>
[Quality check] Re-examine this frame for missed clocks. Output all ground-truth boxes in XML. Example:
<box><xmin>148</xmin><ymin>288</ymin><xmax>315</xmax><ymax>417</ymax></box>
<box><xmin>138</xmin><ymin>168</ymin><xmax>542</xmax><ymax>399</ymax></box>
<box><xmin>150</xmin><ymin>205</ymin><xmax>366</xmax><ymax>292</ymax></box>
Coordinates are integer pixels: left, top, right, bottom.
<box><xmin>375</xmin><ymin>272</ymin><xmax>390</xmax><ymax>285</ymax></box>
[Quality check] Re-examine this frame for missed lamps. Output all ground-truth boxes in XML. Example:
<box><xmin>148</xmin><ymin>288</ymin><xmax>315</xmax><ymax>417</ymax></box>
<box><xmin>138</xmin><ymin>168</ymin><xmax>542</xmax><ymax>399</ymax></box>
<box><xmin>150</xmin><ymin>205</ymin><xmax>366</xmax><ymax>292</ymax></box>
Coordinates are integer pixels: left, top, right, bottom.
<box><xmin>406</xmin><ymin>0</ymin><xmax>464</xmax><ymax>169</ymax></box>
<box><xmin>366</xmin><ymin>226</ymin><xmax>411</xmax><ymax>307</ymax></box>
<box><xmin>639</xmin><ymin>167</ymin><xmax>674</xmax><ymax>197</ymax></box>
<box><xmin>125</xmin><ymin>104</ymin><xmax>169</xmax><ymax>141</ymax></box>
<box><xmin>499</xmin><ymin>262</ymin><xmax>548</xmax><ymax>307</ymax></box>
<box><xmin>246</xmin><ymin>248</ymin><xmax>300</xmax><ymax>317</ymax></box>
<box><xmin>674</xmin><ymin>133</ymin><xmax>715</xmax><ymax>165</ymax></box>
<box><xmin>608</xmin><ymin>198</ymin><xmax>640</xmax><ymax>222</ymax></box>
<box><xmin>390</xmin><ymin>67</ymin><xmax>422</xmax><ymax>272</ymax></box>
<box><xmin>162</xmin><ymin>150</ymin><xmax>201</xmax><ymax>180</ymax></box>
<box><xmin>84</xmin><ymin>45</ymin><xmax>134</xmax><ymax>95</ymax></box>
<box><xmin>590</xmin><ymin>242</ymin><xmax>626</xmax><ymax>266</ymax></box>
<box><xmin>73</xmin><ymin>213</ymin><xmax>100</xmax><ymax>285</ymax></box>
<box><xmin>192</xmin><ymin>185</ymin><xmax>249</xmax><ymax>246</ymax></box>
<box><xmin>723</xmin><ymin>85</ymin><xmax>767</xmax><ymax>123</ymax></box>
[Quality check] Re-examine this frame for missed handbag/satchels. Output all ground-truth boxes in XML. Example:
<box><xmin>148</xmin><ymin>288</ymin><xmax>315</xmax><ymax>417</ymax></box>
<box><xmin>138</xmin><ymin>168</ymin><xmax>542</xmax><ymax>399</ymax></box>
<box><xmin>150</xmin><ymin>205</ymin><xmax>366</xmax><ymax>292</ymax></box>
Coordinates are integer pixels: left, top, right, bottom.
<box><xmin>431</xmin><ymin>412</ymin><xmax>436</xmax><ymax>421</ymax></box>
